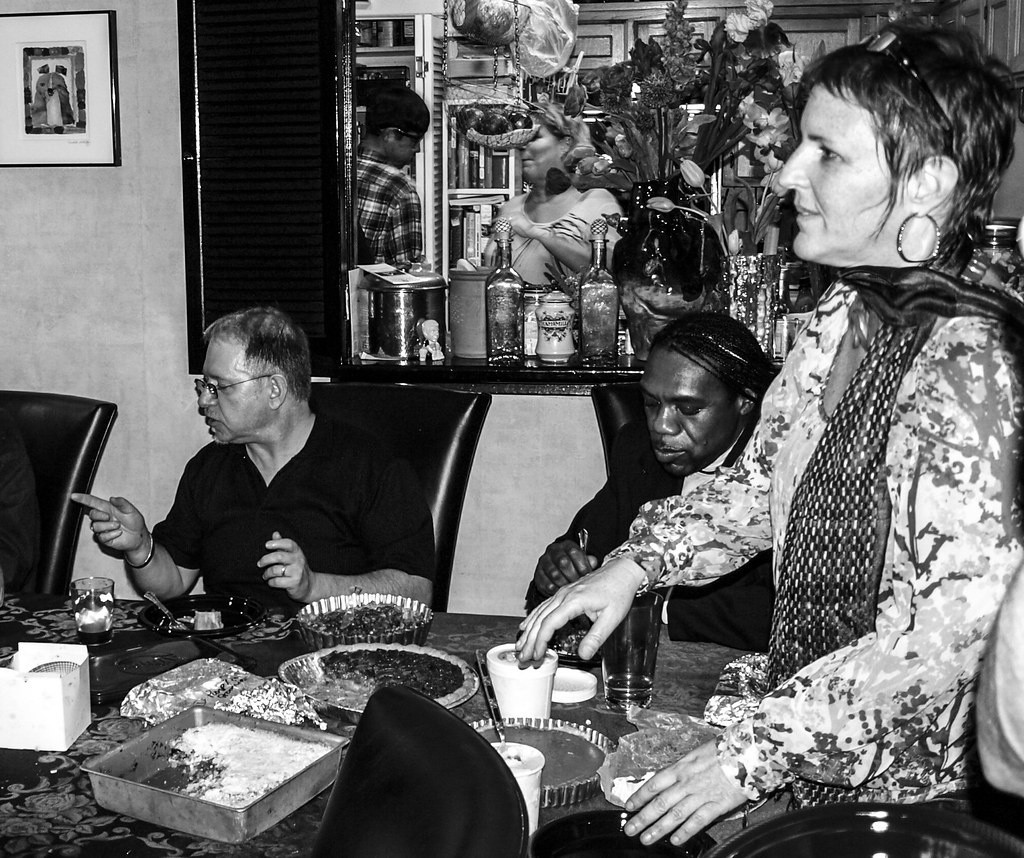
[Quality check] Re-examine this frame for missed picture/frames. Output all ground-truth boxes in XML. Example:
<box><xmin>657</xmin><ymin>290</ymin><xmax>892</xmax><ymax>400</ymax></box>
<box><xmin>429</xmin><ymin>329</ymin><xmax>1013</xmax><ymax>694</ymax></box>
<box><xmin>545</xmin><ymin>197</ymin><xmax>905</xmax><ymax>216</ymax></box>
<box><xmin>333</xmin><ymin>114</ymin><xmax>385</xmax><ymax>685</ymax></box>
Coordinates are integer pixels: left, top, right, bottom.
<box><xmin>0</xmin><ymin>9</ymin><xmax>122</xmax><ymax>167</ymax></box>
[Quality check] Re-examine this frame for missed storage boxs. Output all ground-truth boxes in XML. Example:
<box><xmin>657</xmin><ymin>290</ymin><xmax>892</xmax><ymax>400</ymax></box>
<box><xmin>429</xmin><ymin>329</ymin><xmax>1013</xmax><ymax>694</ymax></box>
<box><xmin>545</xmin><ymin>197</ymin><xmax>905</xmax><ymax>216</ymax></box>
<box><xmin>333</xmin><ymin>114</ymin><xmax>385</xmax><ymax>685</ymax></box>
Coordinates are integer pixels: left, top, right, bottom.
<box><xmin>78</xmin><ymin>704</ymin><xmax>352</xmax><ymax>845</ymax></box>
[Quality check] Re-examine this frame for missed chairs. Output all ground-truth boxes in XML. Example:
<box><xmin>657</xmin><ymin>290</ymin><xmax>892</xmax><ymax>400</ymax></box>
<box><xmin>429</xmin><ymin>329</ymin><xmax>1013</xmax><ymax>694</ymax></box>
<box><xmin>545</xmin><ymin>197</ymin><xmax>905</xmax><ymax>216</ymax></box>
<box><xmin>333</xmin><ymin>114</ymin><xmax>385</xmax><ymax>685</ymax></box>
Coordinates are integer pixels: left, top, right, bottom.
<box><xmin>310</xmin><ymin>382</ymin><xmax>492</xmax><ymax>612</ymax></box>
<box><xmin>0</xmin><ymin>390</ymin><xmax>118</xmax><ymax>595</ymax></box>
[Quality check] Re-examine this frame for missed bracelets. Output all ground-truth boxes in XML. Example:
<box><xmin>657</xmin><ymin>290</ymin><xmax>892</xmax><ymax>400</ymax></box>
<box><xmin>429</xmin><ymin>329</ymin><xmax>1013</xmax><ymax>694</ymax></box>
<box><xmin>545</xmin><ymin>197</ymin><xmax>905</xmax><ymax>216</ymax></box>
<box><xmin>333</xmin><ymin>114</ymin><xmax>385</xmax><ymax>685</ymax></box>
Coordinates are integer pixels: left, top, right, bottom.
<box><xmin>123</xmin><ymin>533</ymin><xmax>155</xmax><ymax>568</ymax></box>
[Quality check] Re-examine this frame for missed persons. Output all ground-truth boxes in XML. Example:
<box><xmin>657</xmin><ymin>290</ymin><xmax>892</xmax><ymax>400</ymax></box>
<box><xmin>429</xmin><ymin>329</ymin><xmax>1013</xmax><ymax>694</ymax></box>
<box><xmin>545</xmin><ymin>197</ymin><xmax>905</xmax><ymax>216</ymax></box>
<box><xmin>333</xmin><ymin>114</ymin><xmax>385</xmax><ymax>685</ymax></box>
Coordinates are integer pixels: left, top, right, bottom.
<box><xmin>481</xmin><ymin>102</ymin><xmax>627</xmax><ymax>284</ymax></box>
<box><xmin>356</xmin><ymin>83</ymin><xmax>433</xmax><ymax>264</ymax></box>
<box><xmin>523</xmin><ymin>311</ymin><xmax>776</xmax><ymax>651</ymax></box>
<box><xmin>69</xmin><ymin>307</ymin><xmax>436</xmax><ymax>615</ymax></box>
<box><xmin>513</xmin><ymin>18</ymin><xmax>1024</xmax><ymax>846</ymax></box>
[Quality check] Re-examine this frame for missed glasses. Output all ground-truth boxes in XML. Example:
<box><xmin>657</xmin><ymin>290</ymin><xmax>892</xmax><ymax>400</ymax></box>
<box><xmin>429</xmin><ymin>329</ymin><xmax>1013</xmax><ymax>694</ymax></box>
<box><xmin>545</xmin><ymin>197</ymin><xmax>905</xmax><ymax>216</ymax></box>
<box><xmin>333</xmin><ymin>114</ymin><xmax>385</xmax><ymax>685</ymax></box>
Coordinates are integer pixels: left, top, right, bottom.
<box><xmin>860</xmin><ymin>30</ymin><xmax>953</xmax><ymax>131</ymax></box>
<box><xmin>381</xmin><ymin>127</ymin><xmax>423</xmax><ymax>145</ymax></box>
<box><xmin>195</xmin><ymin>373</ymin><xmax>273</xmax><ymax>399</ymax></box>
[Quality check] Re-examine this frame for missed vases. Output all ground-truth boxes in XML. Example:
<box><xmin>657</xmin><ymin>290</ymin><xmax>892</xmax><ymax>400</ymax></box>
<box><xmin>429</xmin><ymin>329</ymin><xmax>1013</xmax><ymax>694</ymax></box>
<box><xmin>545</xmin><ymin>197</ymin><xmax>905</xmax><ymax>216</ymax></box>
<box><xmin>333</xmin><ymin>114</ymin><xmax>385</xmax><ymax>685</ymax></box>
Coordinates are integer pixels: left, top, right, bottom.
<box><xmin>611</xmin><ymin>180</ymin><xmax>725</xmax><ymax>360</ymax></box>
<box><xmin>728</xmin><ymin>256</ymin><xmax>773</xmax><ymax>360</ymax></box>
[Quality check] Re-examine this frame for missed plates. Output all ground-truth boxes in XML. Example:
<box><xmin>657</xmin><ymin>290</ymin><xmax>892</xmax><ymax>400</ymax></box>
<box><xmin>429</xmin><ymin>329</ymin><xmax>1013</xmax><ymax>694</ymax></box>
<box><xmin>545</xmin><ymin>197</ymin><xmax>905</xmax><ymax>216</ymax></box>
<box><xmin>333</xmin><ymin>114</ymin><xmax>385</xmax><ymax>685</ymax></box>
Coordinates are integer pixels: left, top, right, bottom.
<box><xmin>528</xmin><ymin>808</ymin><xmax>718</xmax><ymax>858</ymax></box>
<box><xmin>139</xmin><ymin>593</ymin><xmax>267</xmax><ymax>639</ymax></box>
<box><xmin>517</xmin><ymin>630</ymin><xmax>601</xmax><ymax>665</ymax></box>
<box><xmin>278</xmin><ymin>642</ymin><xmax>479</xmax><ymax>725</ymax></box>
<box><xmin>469</xmin><ymin>716</ymin><xmax>616</xmax><ymax>809</ymax></box>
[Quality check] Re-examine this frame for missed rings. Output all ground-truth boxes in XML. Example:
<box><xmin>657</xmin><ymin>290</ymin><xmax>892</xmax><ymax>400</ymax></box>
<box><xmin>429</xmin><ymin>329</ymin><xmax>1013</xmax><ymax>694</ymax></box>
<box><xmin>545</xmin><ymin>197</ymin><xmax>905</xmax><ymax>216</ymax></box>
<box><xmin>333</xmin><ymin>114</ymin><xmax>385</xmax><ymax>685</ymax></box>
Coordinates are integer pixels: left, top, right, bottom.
<box><xmin>282</xmin><ymin>565</ymin><xmax>287</xmax><ymax>576</ymax></box>
<box><xmin>90</xmin><ymin>522</ymin><xmax>94</xmax><ymax>531</ymax></box>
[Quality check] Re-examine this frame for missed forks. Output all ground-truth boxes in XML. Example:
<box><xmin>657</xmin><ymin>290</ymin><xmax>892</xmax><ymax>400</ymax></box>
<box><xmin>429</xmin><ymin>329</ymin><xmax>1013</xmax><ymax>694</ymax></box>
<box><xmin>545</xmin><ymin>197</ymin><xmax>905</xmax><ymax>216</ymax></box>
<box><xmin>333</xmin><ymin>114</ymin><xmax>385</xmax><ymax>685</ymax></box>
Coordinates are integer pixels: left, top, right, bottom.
<box><xmin>144</xmin><ymin>591</ymin><xmax>191</xmax><ymax>631</ymax></box>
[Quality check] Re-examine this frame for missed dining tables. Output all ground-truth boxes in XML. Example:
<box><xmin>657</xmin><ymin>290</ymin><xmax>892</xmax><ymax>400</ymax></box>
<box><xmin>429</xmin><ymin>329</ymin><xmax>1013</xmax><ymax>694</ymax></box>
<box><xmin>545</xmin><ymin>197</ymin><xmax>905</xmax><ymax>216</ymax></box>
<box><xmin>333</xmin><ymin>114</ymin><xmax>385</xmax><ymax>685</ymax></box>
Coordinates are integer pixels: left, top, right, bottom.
<box><xmin>0</xmin><ymin>594</ymin><xmax>797</xmax><ymax>858</ymax></box>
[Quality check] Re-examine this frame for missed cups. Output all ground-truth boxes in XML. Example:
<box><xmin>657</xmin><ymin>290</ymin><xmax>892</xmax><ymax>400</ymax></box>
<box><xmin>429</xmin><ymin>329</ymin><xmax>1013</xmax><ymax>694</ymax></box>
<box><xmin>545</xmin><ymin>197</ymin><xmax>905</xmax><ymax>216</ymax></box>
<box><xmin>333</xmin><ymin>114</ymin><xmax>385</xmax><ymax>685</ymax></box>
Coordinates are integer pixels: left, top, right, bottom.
<box><xmin>600</xmin><ymin>591</ymin><xmax>663</xmax><ymax>713</ymax></box>
<box><xmin>69</xmin><ymin>576</ymin><xmax>115</xmax><ymax>646</ymax></box>
<box><xmin>490</xmin><ymin>742</ymin><xmax>545</xmax><ymax>837</ymax></box>
<box><xmin>486</xmin><ymin>643</ymin><xmax>558</xmax><ymax>720</ymax></box>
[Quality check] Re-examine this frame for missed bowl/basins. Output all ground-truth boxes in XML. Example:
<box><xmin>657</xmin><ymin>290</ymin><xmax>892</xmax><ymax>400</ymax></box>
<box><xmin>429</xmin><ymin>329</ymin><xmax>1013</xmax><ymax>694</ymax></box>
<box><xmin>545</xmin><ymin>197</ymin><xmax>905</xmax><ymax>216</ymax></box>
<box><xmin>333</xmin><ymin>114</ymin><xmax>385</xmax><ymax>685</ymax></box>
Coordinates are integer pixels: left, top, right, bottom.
<box><xmin>295</xmin><ymin>592</ymin><xmax>434</xmax><ymax>652</ymax></box>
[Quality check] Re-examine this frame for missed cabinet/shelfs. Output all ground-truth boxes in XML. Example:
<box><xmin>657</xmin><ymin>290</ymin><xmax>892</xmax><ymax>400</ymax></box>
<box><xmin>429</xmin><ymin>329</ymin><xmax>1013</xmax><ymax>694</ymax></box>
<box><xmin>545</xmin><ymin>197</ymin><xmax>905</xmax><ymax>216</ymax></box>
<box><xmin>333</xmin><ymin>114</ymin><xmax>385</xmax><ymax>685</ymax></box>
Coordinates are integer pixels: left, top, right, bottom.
<box><xmin>544</xmin><ymin>0</ymin><xmax>1024</xmax><ymax>90</ymax></box>
<box><xmin>354</xmin><ymin>13</ymin><xmax>523</xmax><ymax>333</ymax></box>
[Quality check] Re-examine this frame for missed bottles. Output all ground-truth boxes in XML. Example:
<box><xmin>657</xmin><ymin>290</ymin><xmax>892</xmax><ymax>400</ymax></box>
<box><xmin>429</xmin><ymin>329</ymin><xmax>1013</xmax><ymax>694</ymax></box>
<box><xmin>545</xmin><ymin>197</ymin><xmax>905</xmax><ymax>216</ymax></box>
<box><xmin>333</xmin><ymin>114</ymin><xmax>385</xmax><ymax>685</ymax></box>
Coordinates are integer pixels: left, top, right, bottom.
<box><xmin>524</xmin><ymin>295</ymin><xmax>538</xmax><ymax>360</ymax></box>
<box><xmin>486</xmin><ymin>232</ymin><xmax>524</xmax><ymax>368</ymax></box>
<box><xmin>579</xmin><ymin>219</ymin><xmax>619</xmax><ymax>369</ymax></box>
<box><xmin>982</xmin><ymin>225</ymin><xmax>1024</xmax><ymax>303</ymax></box>
<box><xmin>769</xmin><ymin>264</ymin><xmax>814</xmax><ymax>363</ymax></box>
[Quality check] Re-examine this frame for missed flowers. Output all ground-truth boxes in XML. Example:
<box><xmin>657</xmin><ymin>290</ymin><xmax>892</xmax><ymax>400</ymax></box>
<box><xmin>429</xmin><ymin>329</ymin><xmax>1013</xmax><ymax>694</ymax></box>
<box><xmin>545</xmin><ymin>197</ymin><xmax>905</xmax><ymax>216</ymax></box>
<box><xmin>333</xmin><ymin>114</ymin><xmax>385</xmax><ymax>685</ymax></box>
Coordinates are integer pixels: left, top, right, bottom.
<box><xmin>563</xmin><ymin>1</ymin><xmax>807</xmax><ymax>256</ymax></box>
